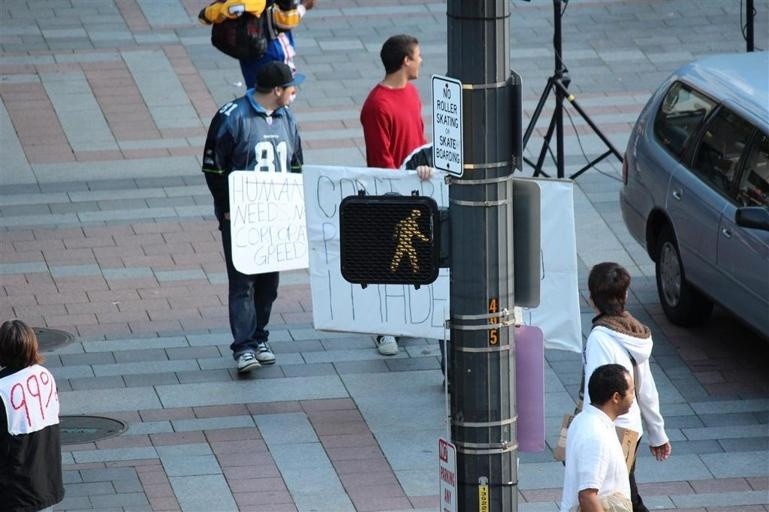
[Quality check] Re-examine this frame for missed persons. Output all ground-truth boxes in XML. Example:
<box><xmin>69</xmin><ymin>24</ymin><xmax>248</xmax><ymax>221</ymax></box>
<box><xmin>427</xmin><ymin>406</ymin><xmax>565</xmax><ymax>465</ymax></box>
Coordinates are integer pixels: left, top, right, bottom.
<box><xmin>359</xmin><ymin>34</ymin><xmax>428</xmax><ymax>353</ymax></box>
<box><xmin>199</xmin><ymin>1</ymin><xmax>314</xmax><ymax>93</ymax></box>
<box><xmin>398</xmin><ymin>142</ymin><xmax>454</xmax><ymax>393</ymax></box>
<box><xmin>0</xmin><ymin>320</ymin><xmax>65</xmax><ymax>512</ymax></box>
<box><xmin>203</xmin><ymin>64</ymin><xmax>304</xmax><ymax>375</ymax></box>
<box><xmin>560</xmin><ymin>365</ymin><xmax>635</xmax><ymax>511</ymax></box>
<box><xmin>576</xmin><ymin>263</ymin><xmax>671</xmax><ymax>512</ymax></box>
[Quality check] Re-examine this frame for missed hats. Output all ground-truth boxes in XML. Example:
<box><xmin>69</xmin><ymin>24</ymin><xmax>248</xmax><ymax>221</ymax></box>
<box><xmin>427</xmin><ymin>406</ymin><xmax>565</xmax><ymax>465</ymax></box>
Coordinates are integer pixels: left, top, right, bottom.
<box><xmin>256</xmin><ymin>61</ymin><xmax>307</xmax><ymax>92</ymax></box>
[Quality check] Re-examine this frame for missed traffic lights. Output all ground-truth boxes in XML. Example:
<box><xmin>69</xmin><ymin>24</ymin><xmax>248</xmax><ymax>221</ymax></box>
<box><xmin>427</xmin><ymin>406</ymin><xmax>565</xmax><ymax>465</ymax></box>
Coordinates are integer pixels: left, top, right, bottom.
<box><xmin>336</xmin><ymin>191</ymin><xmax>439</xmax><ymax>289</ymax></box>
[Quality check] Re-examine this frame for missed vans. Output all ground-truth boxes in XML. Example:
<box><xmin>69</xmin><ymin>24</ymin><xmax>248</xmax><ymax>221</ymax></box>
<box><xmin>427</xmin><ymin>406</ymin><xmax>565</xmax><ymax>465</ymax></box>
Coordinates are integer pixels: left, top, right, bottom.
<box><xmin>611</xmin><ymin>51</ymin><xmax>767</xmax><ymax>350</ymax></box>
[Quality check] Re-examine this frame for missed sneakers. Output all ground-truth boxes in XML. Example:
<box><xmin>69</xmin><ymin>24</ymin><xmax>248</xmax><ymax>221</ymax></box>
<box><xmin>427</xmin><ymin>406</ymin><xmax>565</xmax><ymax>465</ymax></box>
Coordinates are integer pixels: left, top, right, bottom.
<box><xmin>377</xmin><ymin>334</ymin><xmax>399</xmax><ymax>356</ymax></box>
<box><xmin>236</xmin><ymin>352</ymin><xmax>261</xmax><ymax>373</ymax></box>
<box><xmin>255</xmin><ymin>341</ymin><xmax>276</xmax><ymax>365</ymax></box>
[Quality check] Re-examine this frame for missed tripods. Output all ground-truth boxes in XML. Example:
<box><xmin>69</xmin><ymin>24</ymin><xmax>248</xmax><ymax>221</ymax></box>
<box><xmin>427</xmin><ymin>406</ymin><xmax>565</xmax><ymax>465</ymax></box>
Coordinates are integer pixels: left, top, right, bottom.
<box><xmin>523</xmin><ymin>1</ymin><xmax>623</xmax><ymax>177</ymax></box>
